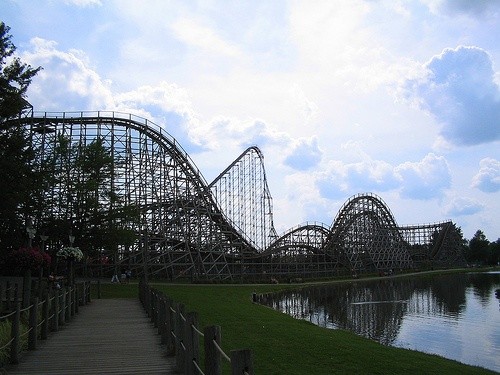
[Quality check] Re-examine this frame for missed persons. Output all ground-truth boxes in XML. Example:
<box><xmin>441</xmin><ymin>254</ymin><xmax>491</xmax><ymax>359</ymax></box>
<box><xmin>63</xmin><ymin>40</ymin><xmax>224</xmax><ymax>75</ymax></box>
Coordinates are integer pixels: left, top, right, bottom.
<box><xmin>463</xmin><ymin>263</ymin><xmax>481</xmax><ymax>268</ymax></box>
<box><xmin>270</xmin><ymin>275</ymin><xmax>305</xmax><ymax>283</ymax></box>
<box><xmin>351</xmin><ymin>269</ymin><xmax>394</xmax><ymax>277</ymax></box>
<box><xmin>111</xmin><ymin>266</ymin><xmax>133</xmax><ymax>281</ymax></box>
